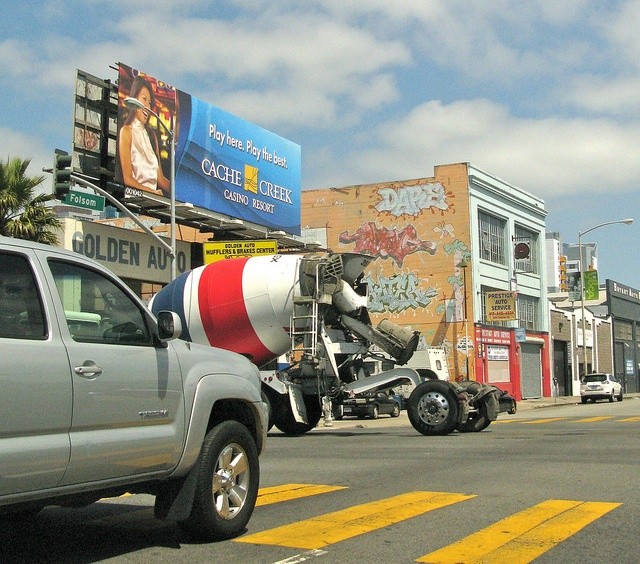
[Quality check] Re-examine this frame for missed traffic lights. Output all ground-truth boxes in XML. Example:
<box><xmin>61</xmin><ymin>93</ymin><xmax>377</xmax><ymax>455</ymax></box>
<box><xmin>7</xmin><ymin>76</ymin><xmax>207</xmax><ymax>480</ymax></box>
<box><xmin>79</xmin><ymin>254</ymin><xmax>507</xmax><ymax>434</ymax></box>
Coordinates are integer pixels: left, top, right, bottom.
<box><xmin>54</xmin><ymin>147</ymin><xmax>72</xmax><ymax>200</ymax></box>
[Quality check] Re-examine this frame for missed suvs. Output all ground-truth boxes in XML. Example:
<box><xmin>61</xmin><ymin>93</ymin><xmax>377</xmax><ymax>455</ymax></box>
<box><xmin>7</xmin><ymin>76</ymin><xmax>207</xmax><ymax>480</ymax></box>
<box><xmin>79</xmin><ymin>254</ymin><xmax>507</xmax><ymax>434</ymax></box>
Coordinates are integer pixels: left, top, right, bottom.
<box><xmin>579</xmin><ymin>370</ymin><xmax>625</xmax><ymax>403</ymax></box>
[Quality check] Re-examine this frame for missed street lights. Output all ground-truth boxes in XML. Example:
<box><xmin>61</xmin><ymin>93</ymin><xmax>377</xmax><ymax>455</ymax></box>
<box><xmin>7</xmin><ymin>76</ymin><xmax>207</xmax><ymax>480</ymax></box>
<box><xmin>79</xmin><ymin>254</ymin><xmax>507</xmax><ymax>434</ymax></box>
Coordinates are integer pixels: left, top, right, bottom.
<box><xmin>575</xmin><ymin>216</ymin><xmax>636</xmax><ymax>378</ymax></box>
<box><xmin>121</xmin><ymin>94</ymin><xmax>177</xmax><ymax>286</ymax></box>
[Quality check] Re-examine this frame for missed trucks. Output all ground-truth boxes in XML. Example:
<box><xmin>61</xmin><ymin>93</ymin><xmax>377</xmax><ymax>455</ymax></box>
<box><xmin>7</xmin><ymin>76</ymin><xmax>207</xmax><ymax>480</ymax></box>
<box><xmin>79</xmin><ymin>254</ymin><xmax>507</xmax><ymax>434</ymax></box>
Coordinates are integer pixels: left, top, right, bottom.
<box><xmin>352</xmin><ymin>344</ymin><xmax>452</xmax><ymax>413</ymax></box>
<box><xmin>141</xmin><ymin>239</ymin><xmax>516</xmax><ymax>437</ymax></box>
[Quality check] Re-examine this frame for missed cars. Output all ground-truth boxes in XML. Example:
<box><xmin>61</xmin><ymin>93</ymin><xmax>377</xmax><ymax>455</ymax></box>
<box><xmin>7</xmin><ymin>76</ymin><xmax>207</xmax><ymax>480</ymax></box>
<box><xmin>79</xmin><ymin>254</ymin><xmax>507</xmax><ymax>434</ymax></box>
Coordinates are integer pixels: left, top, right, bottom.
<box><xmin>0</xmin><ymin>233</ymin><xmax>272</xmax><ymax>540</ymax></box>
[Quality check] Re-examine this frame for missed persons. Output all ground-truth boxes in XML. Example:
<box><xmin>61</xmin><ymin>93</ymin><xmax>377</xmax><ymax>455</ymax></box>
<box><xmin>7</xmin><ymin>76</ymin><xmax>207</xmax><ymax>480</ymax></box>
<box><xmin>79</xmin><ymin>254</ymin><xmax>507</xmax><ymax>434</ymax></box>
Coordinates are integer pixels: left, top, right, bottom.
<box><xmin>119</xmin><ymin>75</ymin><xmax>171</xmax><ymax>196</ymax></box>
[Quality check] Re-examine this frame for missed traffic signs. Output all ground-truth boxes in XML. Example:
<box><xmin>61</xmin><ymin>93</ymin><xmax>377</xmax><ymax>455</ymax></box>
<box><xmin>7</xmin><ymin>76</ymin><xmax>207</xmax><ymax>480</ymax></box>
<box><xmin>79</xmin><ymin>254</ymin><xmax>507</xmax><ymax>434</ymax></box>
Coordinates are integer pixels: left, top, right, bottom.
<box><xmin>60</xmin><ymin>189</ymin><xmax>108</xmax><ymax>212</ymax></box>
